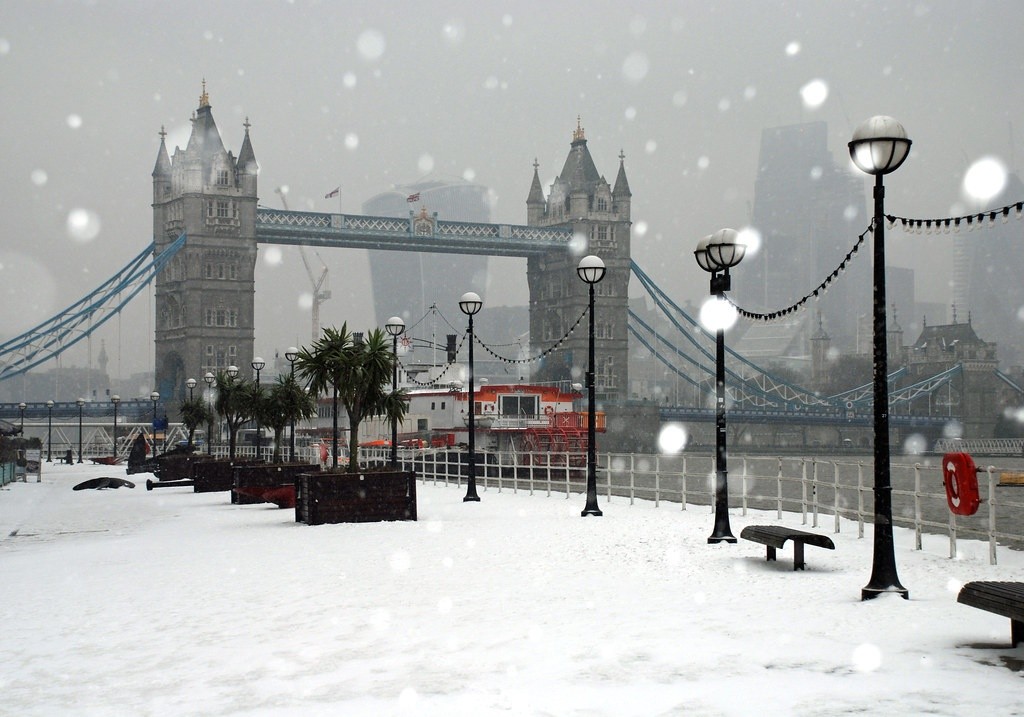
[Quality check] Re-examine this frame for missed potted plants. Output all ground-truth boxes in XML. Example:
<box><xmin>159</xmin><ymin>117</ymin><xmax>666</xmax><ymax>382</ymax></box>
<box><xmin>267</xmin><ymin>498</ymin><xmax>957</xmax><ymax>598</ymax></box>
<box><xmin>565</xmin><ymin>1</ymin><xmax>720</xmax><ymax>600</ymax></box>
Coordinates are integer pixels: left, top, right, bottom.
<box><xmin>157</xmin><ymin>320</ymin><xmax>418</xmax><ymax>525</ymax></box>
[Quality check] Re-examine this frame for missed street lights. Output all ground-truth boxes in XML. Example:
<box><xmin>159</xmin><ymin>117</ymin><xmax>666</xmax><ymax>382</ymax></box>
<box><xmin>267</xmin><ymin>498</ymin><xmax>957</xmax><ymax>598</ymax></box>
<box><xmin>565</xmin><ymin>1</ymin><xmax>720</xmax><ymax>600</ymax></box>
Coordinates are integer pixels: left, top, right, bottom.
<box><xmin>186</xmin><ymin>377</ymin><xmax>196</xmax><ymax>406</ymax></box>
<box><xmin>283</xmin><ymin>347</ymin><xmax>300</xmax><ymax>461</ymax></box>
<box><xmin>76</xmin><ymin>398</ymin><xmax>85</xmax><ymax>464</ymax></box>
<box><xmin>847</xmin><ymin>112</ymin><xmax>915</xmax><ymax>607</ymax></box>
<box><xmin>45</xmin><ymin>400</ymin><xmax>55</xmax><ymax>464</ymax></box>
<box><xmin>111</xmin><ymin>395</ymin><xmax>122</xmax><ymax>461</ymax></box>
<box><xmin>384</xmin><ymin>316</ymin><xmax>406</xmax><ymax>474</ymax></box>
<box><xmin>251</xmin><ymin>357</ymin><xmax>267</xmax><ymax>464</ymax></box>
<box><xmin>19</xmin><ymin>402</ymin><xmax>28</xmax><ymax>437</ymax></box>
<box><xmin>692</xmin><ymin>224</ymin><xmax>748</xmax><ymax>546</ymax></box>
<box><xmin>204</xmin><ymin>371</ymin><xmax>214</xmax><ymax>456</ymax></box>
<box><xmin>456</xmin><ymin>290</ymin><xmax>482</xmax><ymax>504</ymax></box>
<box><xmin>227</xmin><ymin>365</ymin><xmax>240</xmax><ymax>383</ymax></box>
<box><xmin>150</xmin><ymin>390</ymin><xmax>160</xmax><ymax>462</ymax></box>
<box><xmin>578</xmin><ymin>255</ymin><xmax>605</xmax><ymax>515</ymax></box>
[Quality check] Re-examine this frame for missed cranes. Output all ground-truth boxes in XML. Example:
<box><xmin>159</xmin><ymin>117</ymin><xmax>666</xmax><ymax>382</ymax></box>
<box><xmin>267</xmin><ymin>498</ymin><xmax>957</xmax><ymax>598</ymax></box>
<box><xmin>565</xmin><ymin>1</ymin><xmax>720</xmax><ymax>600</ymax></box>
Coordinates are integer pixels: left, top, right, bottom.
<box><xmin>272</xmin><ymin>186</ymin><xmax>331</xmax><ymax>360</ymax></box>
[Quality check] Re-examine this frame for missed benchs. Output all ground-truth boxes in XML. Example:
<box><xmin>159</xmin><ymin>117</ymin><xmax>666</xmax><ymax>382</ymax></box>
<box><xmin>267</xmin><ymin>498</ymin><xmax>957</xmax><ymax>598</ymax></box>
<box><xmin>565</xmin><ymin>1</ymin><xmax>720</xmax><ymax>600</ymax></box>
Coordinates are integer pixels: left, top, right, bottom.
<box><xmin>741</xmin><ymin>525</ymin><xmax>833</xmax><ymax>571</ymax></box>
<box><xmin>958</xmin><ymin>581</ymin><xmax>1023</xmax><ymax>649</ymax></box>
